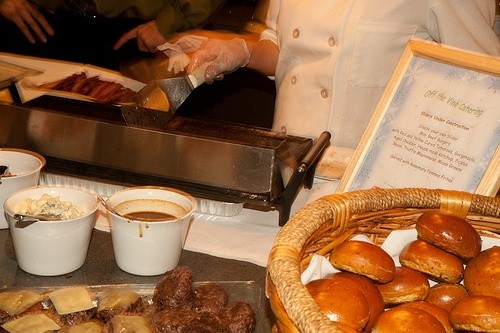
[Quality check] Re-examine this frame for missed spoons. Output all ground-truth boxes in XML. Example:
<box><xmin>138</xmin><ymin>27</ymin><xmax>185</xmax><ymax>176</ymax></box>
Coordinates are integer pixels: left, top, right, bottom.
<box><xmin>14</xmin><ymin>213</ymin><xmax>61</xmax><ymax>222</ymax></box>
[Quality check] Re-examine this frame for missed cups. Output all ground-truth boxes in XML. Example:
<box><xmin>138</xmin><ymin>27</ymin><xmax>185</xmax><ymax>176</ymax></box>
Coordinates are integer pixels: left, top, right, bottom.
<box><xmin>105</xmin><ymin>186</ymin><xmax>197</xmax><ymax>276</ymax></box>
<box><xmin>0</xmin><ymin>147</ymin><xmax>47</xmax><ymax>229</ymax></box>
<box><xmin>3</xmin><ymin>187</ymin><xmax>99</xmax><ymax>276</ymax></box>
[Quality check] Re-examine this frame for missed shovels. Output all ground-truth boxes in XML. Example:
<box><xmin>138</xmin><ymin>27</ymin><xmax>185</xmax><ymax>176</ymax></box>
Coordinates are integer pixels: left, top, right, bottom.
<box><xmin>121</xmin><ymin>62</ymin><xmax>214</xmax><ymax>128</ymax></box>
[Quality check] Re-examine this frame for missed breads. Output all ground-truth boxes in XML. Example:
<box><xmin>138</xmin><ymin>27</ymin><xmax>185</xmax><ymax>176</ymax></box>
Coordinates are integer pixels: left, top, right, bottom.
<box><xmin>303</xmin><ymin>210</ymin><xmax>500</xmax><ymax>333</ymax></box>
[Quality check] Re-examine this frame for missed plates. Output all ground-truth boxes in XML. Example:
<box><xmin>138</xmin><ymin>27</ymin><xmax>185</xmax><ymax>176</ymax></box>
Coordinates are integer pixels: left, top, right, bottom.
<box><xmin>0</xmin><ymin>281</ymin><xmax>272</xmax><ymax>333</ymax></box>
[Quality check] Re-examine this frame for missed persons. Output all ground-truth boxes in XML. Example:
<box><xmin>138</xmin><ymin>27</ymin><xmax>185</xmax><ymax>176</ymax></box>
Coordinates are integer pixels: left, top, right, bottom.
<box><xmin>188</xmin><ymin>0</ymin><xmax>500</xmax><ymax>150</ymax></box>
<box><xmin>0</xmin><ymin>0</ymin><xmax>220</xmax><ymax>74</ymax></box>
